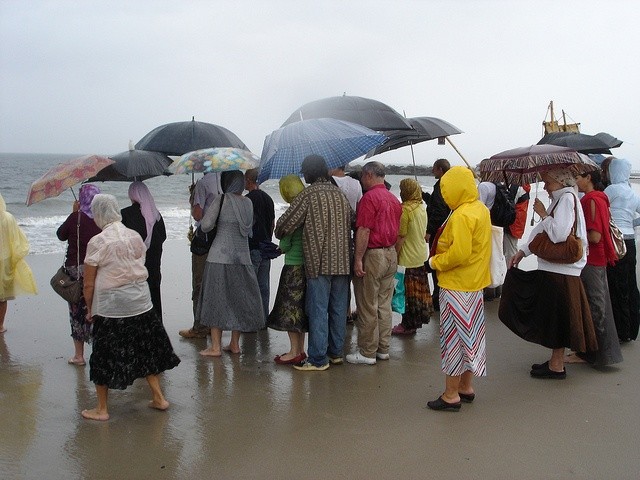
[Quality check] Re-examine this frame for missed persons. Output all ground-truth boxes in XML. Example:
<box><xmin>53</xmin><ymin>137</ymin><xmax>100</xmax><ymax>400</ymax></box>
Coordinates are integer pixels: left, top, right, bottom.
<box><xmin>120</xmin><ymin>182</ymin><xmax>167</xmax><ymax>325</ymax></box>
<box><xmin>560</xmin><ymin>171</ymin><xmax>624</xmax><ymax>369</ymax></box>
<box><xmin>199</xmin><ymin>170</ymin><xmax>267</xmax><ymax>358</ymax></box>
<box><xmin>179</xmin><ymin>171</ymin><xmax>238</xmax><ymax>339</ymax></box>
<box><xmin>265</xmin><ymin>174</ymin><xmax>306</xmax><ymax>365</ymax></box>
<box><xmin>239</xmin><ymin>168</ymin><xmax>282</xmax><ymax>334</ymax></box>
<box><xmin>498</xmin><ymin>166</ymin><xmax>599</xmax><ymax>378</ymax></box>
<box><xmin>345</xmin><ymin>161</ymin><xmax>402</xmax><ymax>365</ymax></box>
<box><xmin>0</xmin><ymin>190</ymin><xmax>38</xmax><ymax>333</ymax></box>
<box><xmin>415</xmin><ymin>159</ymin><xmax>451</xmax><ymax>311</ymax></box>
<box><xmin>599</xmin><ymin>158</ymin><xmax>640</xmax><ymax>344</ymax></box>
<box><xmin>81</xmin><ymin>193</ymin><xmax>182</xmax><ymax>421</ymax></box>
<box><xmin>55</xmin><ymin>184</ymin><xmax>119</xmax><ymax>367</ymax></box>
<box><xmin>385</xmin><ymin>177</ymin><xmax>435</xmax><ymax>335</ymax></box>
<box><xmin>331</xmin><ymin>163</ymin><xmax>355</xmax><ymax>323</ymax></box>
<box><xmin>348</xmin><ymin>163</ymin><xmax>391</xmax><ymax>198</ymax></box>
<box><xmin>600</xmin><ymin>156</ymin><xmax>621</xmax><ymax>191</ymax></box>
<box><xmin>424</xmin><ymin>166</ymin><xmax>492</xmax><ymax>411</ymax></box>
<box><xmin>274</xmin><ymin>156</ymin><xmax>355</xmax><ymax>372</ymax></box>
<box><xmin>477</xmin><ymin>158</ymin><xmax>507</xmax><ymax>299</ymax></box>
<box><xmin>503</xmin><ymin>180</ymin><xmax>531</xmax><ymax>271</ymax></box>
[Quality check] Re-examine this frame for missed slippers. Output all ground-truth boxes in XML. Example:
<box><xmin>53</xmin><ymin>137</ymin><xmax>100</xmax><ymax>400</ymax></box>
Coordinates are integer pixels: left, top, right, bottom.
<box><xmin>68</xmin><ymin>357</ymin><xmax>86</xmax><ymax>366</ymax></box>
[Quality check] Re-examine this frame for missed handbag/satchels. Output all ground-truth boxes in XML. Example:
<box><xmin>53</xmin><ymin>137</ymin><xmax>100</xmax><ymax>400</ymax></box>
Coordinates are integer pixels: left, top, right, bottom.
<box><xmin>50</xmin><ymin>265</ymin><xmax>81</xmax><ymax>305</ymax></box>
<box><xmin>528</xmin><ymin>233</ymin><xmax>583</xmax><ymax>264</ymax></box>
<box><xmin>190</xmin><ymin>224</ymin><xmax>217</xmax><ymax>256</ymax></box>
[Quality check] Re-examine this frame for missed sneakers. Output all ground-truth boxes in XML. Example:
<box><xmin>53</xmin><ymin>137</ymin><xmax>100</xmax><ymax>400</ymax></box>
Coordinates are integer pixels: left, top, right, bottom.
<box><xmin>376</xmin><ymin>352</ymin><xmax>390</xmax><ymax>360</ymax></box>
<box><xmin>392</xmin><ymin>323</ymin><xmax>412</xmax><ymax>335</ymax></box>
<box><xmin>564</xmin><ymin>353</ymin><xmax>587</xmax><ymax>364</ymax></box>
<box><xmin>326</xmin><ymin>352</ymin><xmax>344</xmax><ymax>365</ymax></box>
<box><xmin>530</xmin><ymin>365</ymin><xmax>566</xmax><ymax>379</ymax></box>
<box><xmin>292</xmin><ymin>357</ymin><xmax>330</xmax><ymax>371</ymax></box>
<box><xmin>179</xmin><ymin>328</ymin><xmax>206</xmax><ymax>338</ymax></box>
<box><xmin>532</xmin><ymin>360</ymin><xmax>566</xmax><ymax>373</ymax></box>
<box><xmin>394</xmin><ymin>325</ymin><xmax>416</xmax><ymax>333</ymax></box>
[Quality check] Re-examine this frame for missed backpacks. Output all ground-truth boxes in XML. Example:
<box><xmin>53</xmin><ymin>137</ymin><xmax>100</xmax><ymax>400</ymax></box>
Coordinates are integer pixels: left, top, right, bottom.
<box><xmin>490</xmin><ymin>181</ymin><xmax>516</xmax><ymax>227</ymax></box>
<box><xmin>608</xmin><ymin>220</ymin><xmax>627</xmax><ymax>260</ymax></box>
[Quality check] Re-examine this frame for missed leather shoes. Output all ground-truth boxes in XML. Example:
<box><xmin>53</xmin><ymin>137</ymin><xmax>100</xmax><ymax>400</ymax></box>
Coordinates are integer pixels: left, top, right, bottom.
<box><xmin>443</xmin><ymin>393</ymin><xmax>475</xmax><ymax>403</ymax></box>
<box><xmin>281</xmin><ymin>352</ymin><xmax>307</xmax><ymax>360</ymax></box>
<box><xmin>274</xmin><ymin>355</ymin><xmax>304</xmax><ymax>364</ymax></box>
<box><xmin>345</xmin><ymin>350</ymin><xmax>377</xmax><ymax>365</ymax></box>
<box><xmin>427</xmin><ymin>395</ymin><xmax>462</xmax><ymax>412</ymax></box>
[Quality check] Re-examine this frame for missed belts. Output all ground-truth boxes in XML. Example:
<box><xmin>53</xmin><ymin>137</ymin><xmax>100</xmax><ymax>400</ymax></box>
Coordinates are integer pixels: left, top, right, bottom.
<box><xmin>374</xmin><ymin>246</ymin><xmax>383</xmax><ymax>249</ymax></box>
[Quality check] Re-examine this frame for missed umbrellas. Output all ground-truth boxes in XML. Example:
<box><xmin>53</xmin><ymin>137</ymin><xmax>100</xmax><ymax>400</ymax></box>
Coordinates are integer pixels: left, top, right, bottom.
<box><xmin>279</xmin><ymin>92</ymin><xmax>414</xmax><ymax>131</ymax></box>
<box><xmin>78</xmin><ymin>138</ymin><xmax>180</xmax><ymax>185</ymax></box>
<box><xmin>362</xmin><ymin>109</ymin><xmax>465</xmax><ymax>181</ymax></box>
<box><xmin>23</xmin><ymin>153</ymin><xmax>115</xmax><ymax>207</ymax></box>
<box><xmin>254</xmin><ymin>112</ymin><xmax>390</xmax><ymax>188</ymax></box>
<box><xmin>479</xmin><ymin>144</ymin><xmax>603</xmax><ymax>225</ymax></box>
<box><xmin>591</xmin><ymin>133</ymin><xmax>623</xmax><ymax>148</ymax></box>
<box><xmin>167</xmin><ymin>146</ymin><xmax>262</xmax><ymax>173</ymax></box>
<box><xmin>132</xmin><ymin>115</ymin><xmax>251</xmax><ymax>187</ymax></box>
<box><xmin>535</xmin><ymin>132</ymin><xmax>614</xmax><ymax>156</ymax></box>
